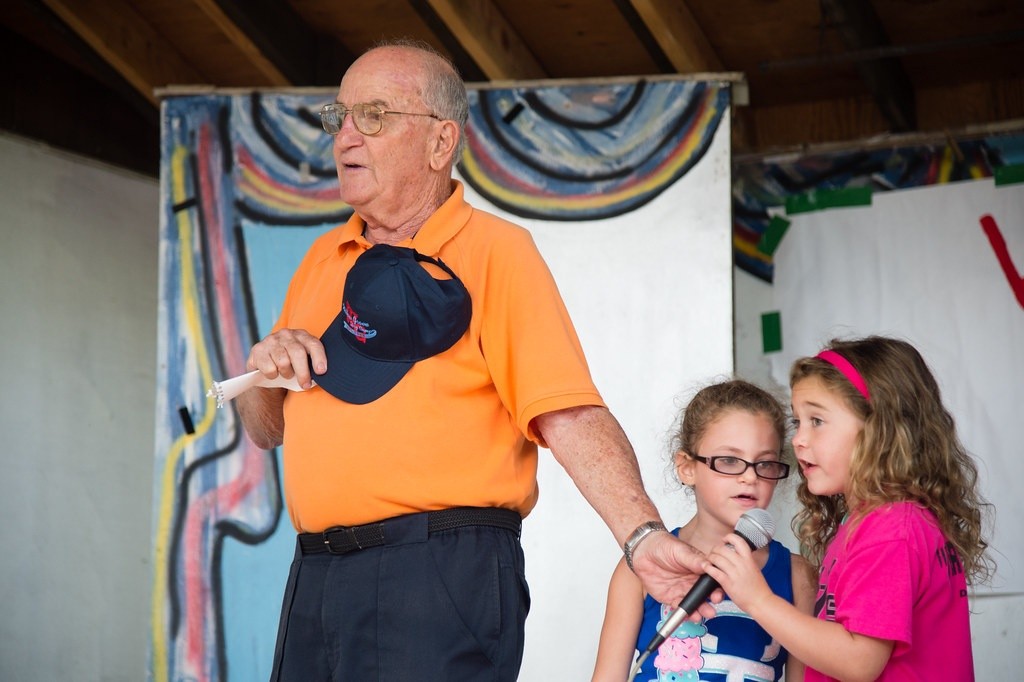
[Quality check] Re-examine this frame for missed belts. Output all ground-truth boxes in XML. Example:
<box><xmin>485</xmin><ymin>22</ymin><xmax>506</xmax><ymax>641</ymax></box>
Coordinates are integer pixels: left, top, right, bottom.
<box><xmin>298</xmin><ymin>508</ymin><xmax>520</xmax><ymax>558</ymax></box>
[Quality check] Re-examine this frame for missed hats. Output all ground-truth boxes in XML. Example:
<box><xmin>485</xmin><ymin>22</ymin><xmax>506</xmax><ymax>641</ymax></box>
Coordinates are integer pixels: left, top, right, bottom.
<box><xmin>307</xmin><ymin>244</ymin><xmax>472</xmax><ymax>405</ymax></box>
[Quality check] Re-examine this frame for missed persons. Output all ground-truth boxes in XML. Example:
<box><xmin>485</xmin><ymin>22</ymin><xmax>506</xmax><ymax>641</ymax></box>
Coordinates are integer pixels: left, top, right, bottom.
<box><xmin>591</xmin><ymin>381</ymin><xmax>820</xmax><ymax>682</ymax></box>
<box><xmin>700</xmin><ymin>335</ymin><xmax>993</xmax><ymax>681</ymax></box>
<box><xmin>235</xmin><ymin>44</ymin><xmax>722</xmax><ymax>681</ymax></box>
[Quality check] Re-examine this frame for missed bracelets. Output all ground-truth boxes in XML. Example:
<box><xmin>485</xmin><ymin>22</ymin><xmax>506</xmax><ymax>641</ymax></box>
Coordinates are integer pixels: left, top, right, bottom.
<box><xmin>624</xmin><ymin>521</ymin><xmax>667</xmax><ymax>569</ymax></box>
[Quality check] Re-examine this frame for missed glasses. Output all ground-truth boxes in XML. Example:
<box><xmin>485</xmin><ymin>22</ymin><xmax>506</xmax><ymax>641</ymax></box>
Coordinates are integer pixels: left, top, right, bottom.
<box><xmin>682</xmin><ymin>449</ymin><xmax>791</xmax><ymax>480</ymax></box>
<box><xmin>319</xmin><ymin>102</ymin><xmax>447</xmax><ymax>135</ymax></box>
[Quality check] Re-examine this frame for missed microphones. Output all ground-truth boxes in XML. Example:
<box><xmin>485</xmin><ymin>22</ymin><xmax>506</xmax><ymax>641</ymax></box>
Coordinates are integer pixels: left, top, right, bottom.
<box><xmin>648</xmin><ymin>508</ymin><xmax>776</xmax><ymax>652</ymax></box>
